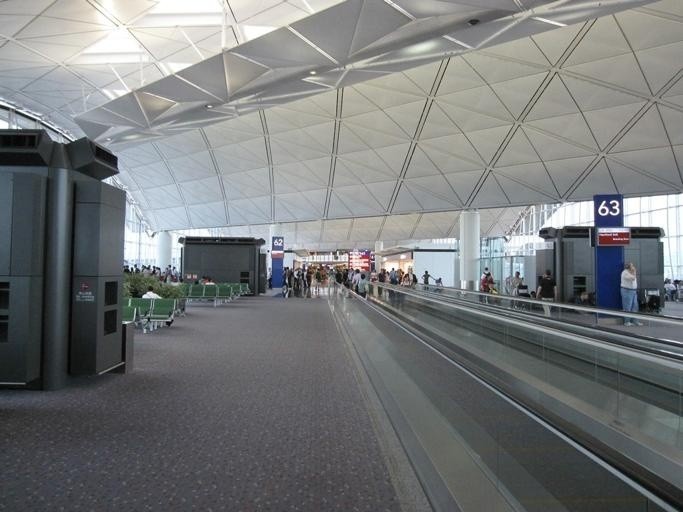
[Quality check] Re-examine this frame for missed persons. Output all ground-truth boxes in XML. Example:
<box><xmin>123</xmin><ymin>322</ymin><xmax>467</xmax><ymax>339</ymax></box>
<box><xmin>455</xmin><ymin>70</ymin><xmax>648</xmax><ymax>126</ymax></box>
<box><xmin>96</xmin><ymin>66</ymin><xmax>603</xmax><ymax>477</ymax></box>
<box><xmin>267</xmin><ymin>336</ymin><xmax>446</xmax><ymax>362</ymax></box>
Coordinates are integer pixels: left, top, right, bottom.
<box><xmin>619</xmin><ymin>262</ymin><xmax>644</xmax><ymax>327</ymax></box>
<box><xmin>435</xmin><ymin>278</ymin><xmax>441</xmax><ymax>287</ymax></box>
<box><xmin>479</xmin><ymin>267</ymin><xmax>494</xmax><ymax>285</ymax></box>
<box><xmin>663</xmin><ymin>278</ymin><xmax>669</xmax><ymax>285</ymax></box>
<box><xmin>122</xmin><ymin>263</ymin><xmax>215</xmax><ymax>285</ymax></box>
<box><xmin>533</xmin><ymin>269</ymin><xmax>558</xmax><ymax>318</ymax></box>
<box><xmin>663</xmin><ymin>278</ymin><xmax>677</xmax><ymax>302</ymax></box>
<box><xmin>140</xmin><ymin>286</ymin><xmax>163</xmax><ymax>299</ymax></box>
<box><xmin>509</xmin><ymin>271</ymin><xmax>522</xmax><ymax>310</ymax></box>
<box><xmin>421</xmin><ymin>270</ymin><xmax>434</xmax><ymax>290</ymax></box>
<box><xmin>282</xmin><ymin>266</ymin><xmax>417</xmax><ymax>305</ymax></box>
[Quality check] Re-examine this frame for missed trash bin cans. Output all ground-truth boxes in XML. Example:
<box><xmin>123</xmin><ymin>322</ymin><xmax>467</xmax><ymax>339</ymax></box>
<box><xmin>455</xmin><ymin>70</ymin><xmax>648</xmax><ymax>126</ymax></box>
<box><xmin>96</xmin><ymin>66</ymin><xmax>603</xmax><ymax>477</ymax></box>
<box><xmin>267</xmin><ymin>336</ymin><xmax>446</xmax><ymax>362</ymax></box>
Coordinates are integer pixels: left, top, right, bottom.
<box><xmin>121</xmin><ymin>320</ymin><xmax>135</xmax><ymax>374</ymax></box>
<box><xmin>573</xmin><ymin>276</ymin><xmax>587</xmax><ymax>297</ymax></box>
<box><xmin>467</xmin><ymin>281</ymin><xmax>474</xmax><ymax>291</ymax></box>
<box><xmin>639</xmin><ymin>287</ymin><xmax>660</xmax><ymax>313</ymax></box>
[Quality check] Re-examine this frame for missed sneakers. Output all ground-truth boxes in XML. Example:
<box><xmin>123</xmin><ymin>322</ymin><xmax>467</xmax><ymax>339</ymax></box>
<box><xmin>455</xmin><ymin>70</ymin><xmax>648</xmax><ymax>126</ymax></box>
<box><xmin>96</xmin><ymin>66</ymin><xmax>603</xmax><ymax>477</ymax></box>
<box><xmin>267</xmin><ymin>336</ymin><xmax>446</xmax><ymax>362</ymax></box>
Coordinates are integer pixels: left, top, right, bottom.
<box><xmin>624</xmin><ymin>321</ymin><xmax>644</xmax><ymax>327</ymax></box>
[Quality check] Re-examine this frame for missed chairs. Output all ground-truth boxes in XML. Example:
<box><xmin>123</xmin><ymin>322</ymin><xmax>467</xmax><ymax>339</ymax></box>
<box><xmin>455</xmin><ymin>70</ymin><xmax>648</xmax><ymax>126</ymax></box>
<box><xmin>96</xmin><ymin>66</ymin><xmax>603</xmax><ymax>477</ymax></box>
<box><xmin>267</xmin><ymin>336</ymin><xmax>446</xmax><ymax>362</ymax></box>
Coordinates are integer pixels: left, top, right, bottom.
<box><xmin>122</xmin><ymin>282</ymin><xmax>252</xmax><ymax>334</ymax></box>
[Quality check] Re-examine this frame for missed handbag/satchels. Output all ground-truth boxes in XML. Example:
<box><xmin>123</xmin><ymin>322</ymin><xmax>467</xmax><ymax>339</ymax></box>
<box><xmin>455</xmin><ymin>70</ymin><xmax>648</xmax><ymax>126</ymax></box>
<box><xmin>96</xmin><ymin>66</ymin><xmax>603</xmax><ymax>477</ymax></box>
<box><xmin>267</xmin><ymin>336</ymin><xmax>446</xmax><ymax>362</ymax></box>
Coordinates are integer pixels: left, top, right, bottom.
<box><xmin>355</xmin><ymin>285</ymin><xmax>358</xmax><ymax>292</ymax></box>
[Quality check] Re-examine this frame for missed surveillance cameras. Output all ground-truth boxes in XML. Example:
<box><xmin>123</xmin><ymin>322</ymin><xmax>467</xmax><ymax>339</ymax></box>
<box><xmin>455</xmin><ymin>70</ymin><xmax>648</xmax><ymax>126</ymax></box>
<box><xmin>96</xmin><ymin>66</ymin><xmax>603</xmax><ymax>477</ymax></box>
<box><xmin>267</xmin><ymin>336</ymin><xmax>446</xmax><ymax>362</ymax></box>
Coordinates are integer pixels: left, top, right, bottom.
<box><xmin>468</xmin><ymin>19</ymin><xmax>480</xmax><ymax>25</ymax></box>
